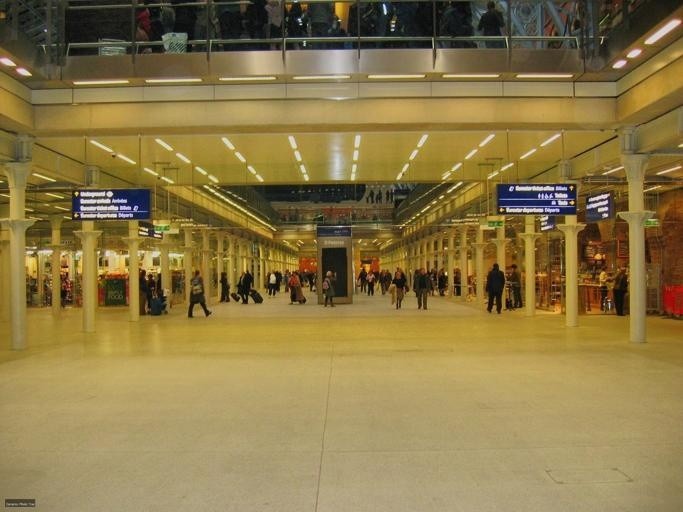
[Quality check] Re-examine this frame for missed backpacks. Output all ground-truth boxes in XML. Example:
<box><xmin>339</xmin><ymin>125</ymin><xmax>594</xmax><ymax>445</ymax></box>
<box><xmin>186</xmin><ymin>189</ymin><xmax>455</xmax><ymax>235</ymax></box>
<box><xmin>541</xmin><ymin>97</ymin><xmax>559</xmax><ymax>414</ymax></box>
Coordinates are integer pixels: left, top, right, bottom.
<box><xmin>288</xmin><ymin>274</ymin><xmax>297</xmax><ymax>287</ymax></box>
<box><xmin>322</xmin><ymin>280</ymin><xmax>330</xmax><ymax>289</ymax></box>
<box><xmin>192</xmin><ymin>279</ymin><xmax>202</xmax><ymax>295</ymax></box>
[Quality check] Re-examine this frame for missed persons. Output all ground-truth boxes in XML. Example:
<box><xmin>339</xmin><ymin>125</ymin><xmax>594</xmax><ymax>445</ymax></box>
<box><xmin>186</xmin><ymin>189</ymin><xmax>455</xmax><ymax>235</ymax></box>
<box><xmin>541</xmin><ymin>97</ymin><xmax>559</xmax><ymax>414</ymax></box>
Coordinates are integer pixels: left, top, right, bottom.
<box><xmin>610</xmin><ymin>267</ymin><xmax>628</xmax><ymax>316</ymax></box>
<box><xmin>187</xmin><ymin>270</ymin><xmax>213</xmax><ymax>318</ymax></box>
<box><xmin>220</xmin><ymin>268</ymin><xmax>317</xmax><ymax>304</ymax></box>
<box><xmin>357</xmin><ymin>267</ymin><xmax>461</xmax><ymax>310</ymax></box>
<box><xmin>365</xmin><ymin>189</ymin><xmax>395</xmax><ymax>205</ymax></box>
<box><xmin>599</xmin><ymin>266</ymin><xmax>609</xmax><ymax>312</ymax></box>
<box><xmin>139</xmin><ymin>269</ymin><xmax>169</xmax><ymax>315</ymax></box>
<box><xmin>486</xmin><ymin>263</ymin><xmax>505</xmax><ymax>313</ymax></box>
<box><xmin>324</xmin><ymin>271</ymin><xmax>337</xmax><ymax>307</ymax></box>
<box><xmin>133</xmin><ymin>1</ymin><xmax>507</xmax><ymax>54</ymax></box>
<box><xmin>506</xmin><ymin>264</ymin><xmax>523</xmax><ymax>308</ymax></box>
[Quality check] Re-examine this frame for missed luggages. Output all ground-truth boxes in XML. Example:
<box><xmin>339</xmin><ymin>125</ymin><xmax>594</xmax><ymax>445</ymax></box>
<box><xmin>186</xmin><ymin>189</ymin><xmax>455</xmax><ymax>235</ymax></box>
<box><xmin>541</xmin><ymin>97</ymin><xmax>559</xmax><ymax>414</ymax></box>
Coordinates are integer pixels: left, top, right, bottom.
<box><xmin>250</xmin><ymin>289</ymin><xmax>263</xmax><ymax>304</ymax></box>
